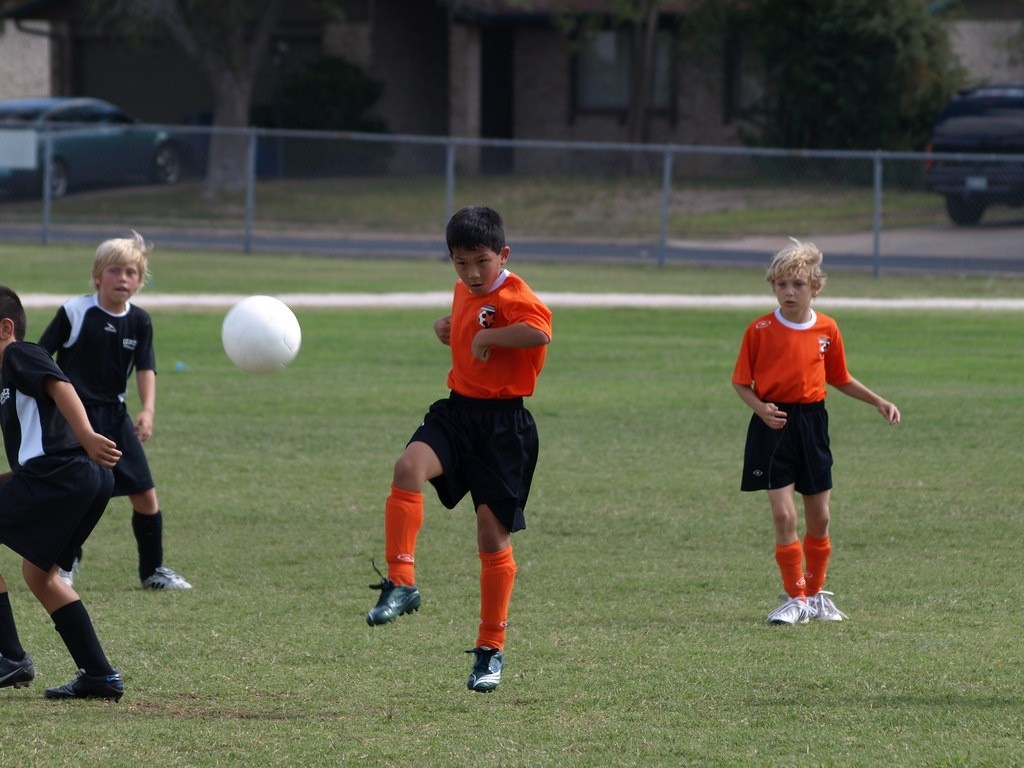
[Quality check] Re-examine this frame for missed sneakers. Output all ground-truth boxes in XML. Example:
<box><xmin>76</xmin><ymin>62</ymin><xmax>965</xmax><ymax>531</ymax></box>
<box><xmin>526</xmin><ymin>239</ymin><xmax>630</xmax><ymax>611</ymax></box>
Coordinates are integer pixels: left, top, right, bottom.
<box><xmin>766</xmin><ymin>596</ymin><xmax>819</xmax><ymax>627</ymax></box>
<box><xmin>0</xmin><ymin>650</ymin><xmax>35</xmax><ymax>688</ymax></box>
<box><xmin>59</xmin><ymin>557</ymin><xmax>81</xmax><ymax>587</ymax></box>
<box><xmin>143</xmin><ymin>566</ymin><xmax>193</xmax><ymax>590</ymax></box>
<box><xmin>365</xmin><ymin>553</ymin><xmax>422</xmax><ymax>626</ymax></box>
<box><xmin>44</xmin><ymin>668</ymin><xmax>125</xmax><ymax>701</ymax></box>
<box><xmin>465</xmin><ymin>645</ymin><xmax>505</xmax><ymax>694</ymax></box>
<box><xmin>811</xmin><ymin>589</ymin><xmax>850</xmax><ymax>622</ymax></box>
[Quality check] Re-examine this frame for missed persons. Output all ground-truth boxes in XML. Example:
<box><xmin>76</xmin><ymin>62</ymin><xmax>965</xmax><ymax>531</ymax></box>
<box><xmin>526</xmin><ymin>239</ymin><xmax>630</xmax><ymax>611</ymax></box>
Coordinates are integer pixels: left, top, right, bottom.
<box><xmin>0</xmin><ymin>284</ymin><xmax>125</xmax><ymax>702</ymax></box>
<box><xmin>36</xmin><ymin>230</ymin><xmax>194</xmax><ymax>591</ymax></box>
<box><xmin>365</xmin><ymin>205</ymin><xmax>553</xmax><ymax>693</ymax></box>
<box><xmin>732</xmin><ymin>237</ymin><xmax>900</xmax><ymax>625</ymax></box>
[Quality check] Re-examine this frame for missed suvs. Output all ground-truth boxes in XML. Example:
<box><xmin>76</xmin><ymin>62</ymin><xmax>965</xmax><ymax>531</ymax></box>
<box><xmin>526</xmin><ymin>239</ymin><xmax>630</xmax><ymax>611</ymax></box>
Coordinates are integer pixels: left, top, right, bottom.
<box><xmin>920</xmin><ymin>82</ymin><xmax>1024</xmax><ymax>225</ymax></box>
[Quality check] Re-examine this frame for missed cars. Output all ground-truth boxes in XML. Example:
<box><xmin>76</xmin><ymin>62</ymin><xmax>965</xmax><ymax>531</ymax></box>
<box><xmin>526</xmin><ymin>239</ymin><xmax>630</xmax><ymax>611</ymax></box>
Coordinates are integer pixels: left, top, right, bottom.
<box><xmin>0</xmin><ymin>96</ymin><xmax>188</xmax><ymax>201</ymax></box>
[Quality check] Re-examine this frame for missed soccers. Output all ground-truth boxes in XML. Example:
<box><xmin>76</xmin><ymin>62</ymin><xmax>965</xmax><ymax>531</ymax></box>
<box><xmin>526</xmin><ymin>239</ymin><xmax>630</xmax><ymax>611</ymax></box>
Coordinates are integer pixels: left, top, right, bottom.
<box><xmin>219</xmin><ymin>295</ymin><xmax>304</xmax><ymax>372</ymax></box>
